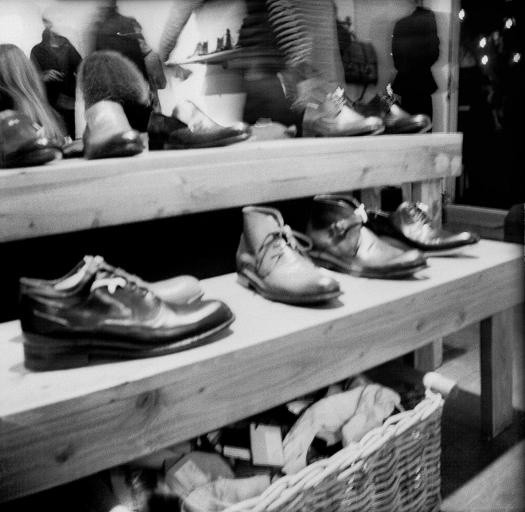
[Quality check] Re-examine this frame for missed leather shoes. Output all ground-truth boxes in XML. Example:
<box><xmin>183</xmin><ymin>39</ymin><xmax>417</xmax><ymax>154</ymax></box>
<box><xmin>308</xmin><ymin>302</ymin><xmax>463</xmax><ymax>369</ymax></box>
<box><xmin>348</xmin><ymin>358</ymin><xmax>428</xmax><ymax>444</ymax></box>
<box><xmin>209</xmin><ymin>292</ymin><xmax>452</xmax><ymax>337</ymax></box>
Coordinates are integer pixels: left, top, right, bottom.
<box><xmin>147</xmin><ymin>100</ymin><xmax>253</xmax><ymax>151</ymax></box>
<box><xmin>83</xmin><ymin>101</ymin><xmax>144</xmax><ymax>159</ymax></box>
<box><xmin>17</xmin><ymin>253</ymin><xmax>235</xmax><ymax>370</ymax></box>
<box><xmin>364</xmin><ymin>199</ymin><xmax>480</xmax><ymax>258</ymax></box>
<box><xmin>0</xmin><ymin>110</ymin><xmax>56</xmax><ymax>168</ymax></box>
<box><xmin>302</xmin><ymin>93</ymin><xmax>386</xmax><ymax>136</ymax></box>
<box><xmin>353</xmin><ymin>93</ymin><xmax>432</xmax><ymax>134</ymax></box>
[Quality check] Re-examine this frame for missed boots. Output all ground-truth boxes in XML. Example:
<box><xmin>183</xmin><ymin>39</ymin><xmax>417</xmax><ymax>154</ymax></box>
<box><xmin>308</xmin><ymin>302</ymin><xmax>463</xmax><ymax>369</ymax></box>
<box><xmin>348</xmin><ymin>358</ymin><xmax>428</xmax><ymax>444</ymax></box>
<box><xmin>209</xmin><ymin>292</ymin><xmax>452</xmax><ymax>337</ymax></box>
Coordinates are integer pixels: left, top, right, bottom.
<box><xmin>303</xmin><ymin>192</ymin><xmax>428</xmax><ymax>276</ymax></box>
<box><xmin>236</xmin><ymin>205</ymin><xmax>342</xmax><ymax>303</ymax></box>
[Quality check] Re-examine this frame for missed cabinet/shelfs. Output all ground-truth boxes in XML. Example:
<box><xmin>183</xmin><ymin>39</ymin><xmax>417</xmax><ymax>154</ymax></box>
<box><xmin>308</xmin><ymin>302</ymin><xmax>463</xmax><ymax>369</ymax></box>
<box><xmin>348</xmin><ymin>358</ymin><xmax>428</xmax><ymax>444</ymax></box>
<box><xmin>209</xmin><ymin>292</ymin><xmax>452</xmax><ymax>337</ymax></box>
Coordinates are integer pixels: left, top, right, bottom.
<box><xmin>1</xmin><ymin>131</ymin><xmax>524</xmax><ymax>511</ymax></box>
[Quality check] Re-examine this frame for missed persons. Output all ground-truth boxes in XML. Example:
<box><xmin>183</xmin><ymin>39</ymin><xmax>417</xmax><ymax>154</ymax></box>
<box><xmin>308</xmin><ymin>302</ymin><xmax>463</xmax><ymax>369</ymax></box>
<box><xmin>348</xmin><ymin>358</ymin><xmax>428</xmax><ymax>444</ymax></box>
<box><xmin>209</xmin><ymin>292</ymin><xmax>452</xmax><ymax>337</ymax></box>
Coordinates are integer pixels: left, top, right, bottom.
<box><xmin>126</xmin><ymin>16</ymin><xmax>168</xmax><ymax>117</ymax></box>
<box><xmin>0</xmin><ymin>43</ymin><xmax>70</xmax><ymax>145</ymax></box>
<box><xmin>388</xmin><ymin>0</ymin><xmax>440</xmax><ymax>132</ymax></box>
<box><xmin>83</xmin><ymin>0</ymin><xmax>160</xmax><ymax>88</ymax></box>
<box><xmin>29</xmin><ymin>8</ymin><xmax>86</xmax><ymax>140</ymax></box>
<box><xmin>76</xmin><ymin>49</ymin><xmax>152</xmax><ymax>132</ymax></box>
<box><xmin>240</xmin><ymin>18</ymin><xmax>356</xmax><ymax>137</ymax></box>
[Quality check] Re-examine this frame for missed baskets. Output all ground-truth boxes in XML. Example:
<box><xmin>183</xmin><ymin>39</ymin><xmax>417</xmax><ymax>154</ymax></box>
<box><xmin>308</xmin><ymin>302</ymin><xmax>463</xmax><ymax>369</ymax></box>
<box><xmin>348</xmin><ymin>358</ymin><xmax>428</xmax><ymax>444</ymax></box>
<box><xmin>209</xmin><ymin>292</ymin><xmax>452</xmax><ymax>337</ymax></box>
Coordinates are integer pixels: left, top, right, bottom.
<box><xmin>102</xmin><ymin>365</ymin><xmax>445</xmax><ymax>512</ymax></box>
